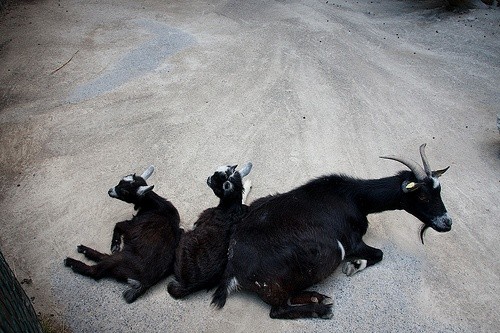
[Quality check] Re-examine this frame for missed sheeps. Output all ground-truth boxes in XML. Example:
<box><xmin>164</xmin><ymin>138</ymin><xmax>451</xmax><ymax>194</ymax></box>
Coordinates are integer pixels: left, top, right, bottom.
<box><xmin>209</xmin><ymin>143</ymin><xmax>454</xmax><ymax>320</ymax></box>
<box><xmin>166</xmin><ymin>162</ymin><xmax>253</xmax><ymax>300</ymax></box>
<box><xmin>64</xmin><ymin>165</ymin><xmax>181</xmax><ymax>304</ymax></box>
